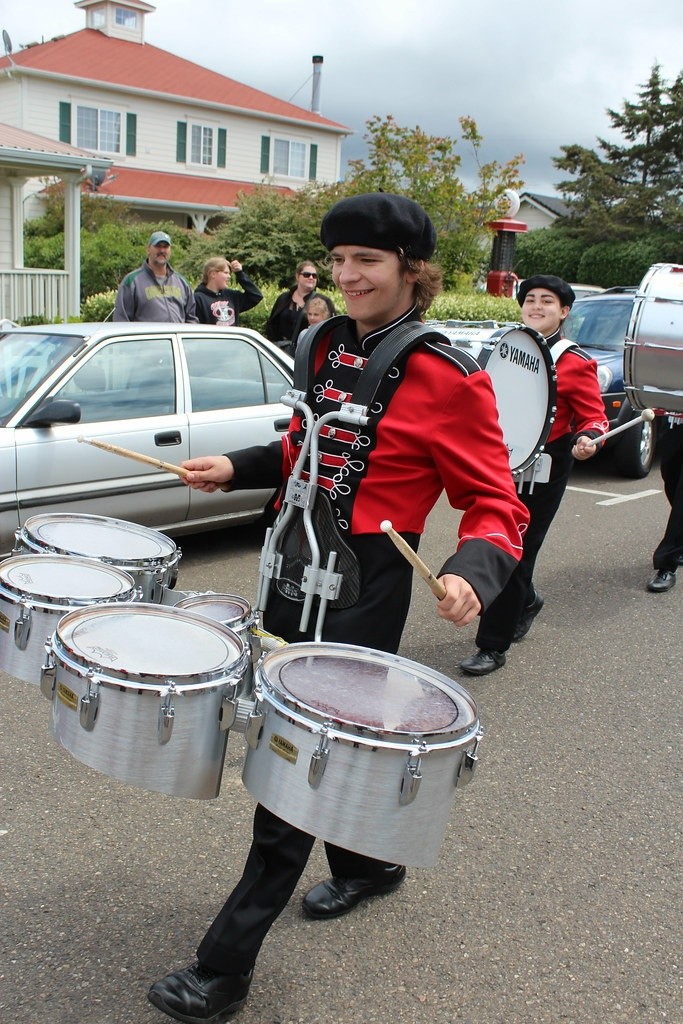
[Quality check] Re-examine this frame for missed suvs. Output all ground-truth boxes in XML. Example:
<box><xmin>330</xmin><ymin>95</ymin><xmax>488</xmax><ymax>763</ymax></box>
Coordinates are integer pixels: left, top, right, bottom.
<box><xmin>562</xmin><ymin>286</ymin><xmax>654</xmax><ymax>479</ymax></box>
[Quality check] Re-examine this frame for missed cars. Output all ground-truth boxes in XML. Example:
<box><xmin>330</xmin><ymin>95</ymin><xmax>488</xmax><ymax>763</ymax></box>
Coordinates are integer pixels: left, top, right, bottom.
<box><xmin>0</xmin><ymin>321</ymin><xmax>297</xmax><ymax>558</ymax></box>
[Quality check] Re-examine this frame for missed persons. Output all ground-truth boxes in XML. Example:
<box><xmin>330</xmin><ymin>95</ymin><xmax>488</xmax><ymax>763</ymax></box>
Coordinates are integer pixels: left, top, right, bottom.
<box><xmin>267</xmin><ymin>261</ymin><xmax>337</xmax><ymax>360</ymax></box>
<box><xmin>295</xmin><ymin>297</ymin><xmax>333</xmax><ymax>356</ymax></box>
<box><xmin>648</xmin><ymin>409</ymin><xmax>683</xmax><ymax>592</ymax></box>
<box><xmin>193</xmin><ymin>257</ymin><xmax>263</xmax><ymax>327</ymax></box>
<box><xmin>113</xmin><ymin>231</ymin><xmax>200</xmax><ymax>323</ymax></box>
<box><xmin>460</xmin><ymin>275</ymin><xmax>610</xmax><ymax>676</ymax></box>
<box><xmin>147</xmin><ymin>188</ymin><xmax>530</xmax><ymax>1024</ymax></box>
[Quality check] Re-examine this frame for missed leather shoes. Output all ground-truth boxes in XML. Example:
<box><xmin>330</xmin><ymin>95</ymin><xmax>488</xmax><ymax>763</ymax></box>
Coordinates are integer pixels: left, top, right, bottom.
<box><xmin>648</xmin><ymin>570</ymin><xmax>676</xmax><ymax>591</ymax></box>
<box><xmin>460</xmin><ymin>650</ymin><xmax>506</xmax><ymax>675</ymax></box>
<box><xmin>301</xmin><ymin>865</ymin><xmax>407</xmax><ymax>918</ymax></box>
<box><xmin>147</xmin><ymin>960</ymin><xmax>255</xmax><ymax>1024</ymax></box>
<box><xmin>678</xmin><ymin>555</ymin><xmax>683</xmax><ymax>565</ymax></box>
<box><xmin>513</xmin><ymin>591</ymin><xmax>544</xmax><ymax>642</ymax></box>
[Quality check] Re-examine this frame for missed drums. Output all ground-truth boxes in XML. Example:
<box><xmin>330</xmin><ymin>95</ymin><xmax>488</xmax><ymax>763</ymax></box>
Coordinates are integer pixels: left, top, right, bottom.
<box><xmin>620</xmin><ymin>261</ymin><xmax>683</xmax><ymax>418</ymax></box>
<box><xmin>423</xmin><ymin>318</ymin><xmax>560</xmax><ymax>478</ymax></box>
<box><xmin>240</xmin><ymin>639</ymin><xmax>481</xmax><ymax>870</ymax></box>
<box><xmin>50</xmin><ymin>601</ymin><xmax>250</xmax><ymax>805</ymax></box>
<box><xmin>172</xmin><ymin>592</ymin><xmax>254</xmax><ymax>700</ymax></box>
<box><xmin>23</xmin><ymin>511</ymin><xmax>177</xmax><ymax>605</ymax></box>
<box><xmin>0</xmin><ymin>552</ymin><xmax>137</xmax><ymax>687</ymax></box>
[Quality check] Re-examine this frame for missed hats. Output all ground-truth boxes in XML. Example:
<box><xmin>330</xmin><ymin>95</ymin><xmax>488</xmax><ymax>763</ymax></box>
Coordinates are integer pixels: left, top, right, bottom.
<box><xmin>312</xmin><ymin>293</ymin><xmax>337</xmax><ymax>318</ymax></box>
<box><xmin>321</xmin><ymin>188</ymin><xmax>437</xmax><ymax>262</ymax></box>
<box><xmin>149</xmin><ymin>231</ymin><xmax>170</xmax><ymax>247</ymax></box>
<box><xmin>517</xmin><ymin>275</ymin><xmax>576</xmax><ymax>308</ymax></box>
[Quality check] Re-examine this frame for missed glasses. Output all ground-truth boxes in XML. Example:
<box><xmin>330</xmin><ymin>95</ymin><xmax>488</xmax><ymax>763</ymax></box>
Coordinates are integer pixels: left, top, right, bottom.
<box><xmin>299</xmin><ymin>272</ymin><xmax>317</xmax><ymax>279</ymax></box>
